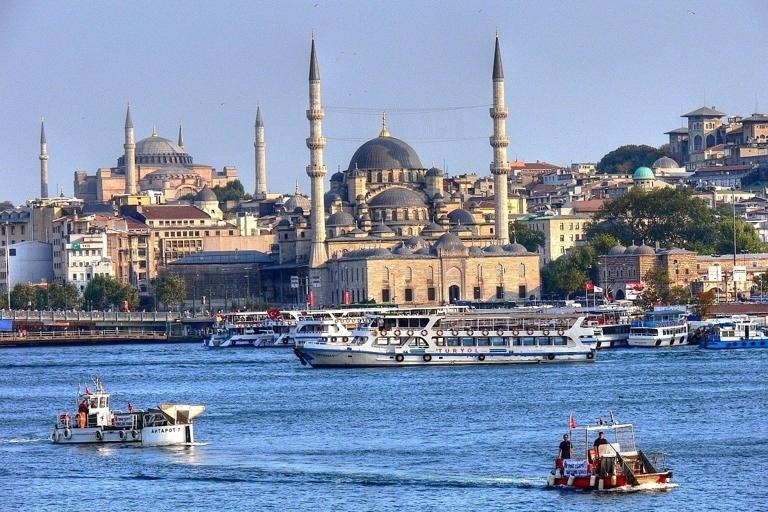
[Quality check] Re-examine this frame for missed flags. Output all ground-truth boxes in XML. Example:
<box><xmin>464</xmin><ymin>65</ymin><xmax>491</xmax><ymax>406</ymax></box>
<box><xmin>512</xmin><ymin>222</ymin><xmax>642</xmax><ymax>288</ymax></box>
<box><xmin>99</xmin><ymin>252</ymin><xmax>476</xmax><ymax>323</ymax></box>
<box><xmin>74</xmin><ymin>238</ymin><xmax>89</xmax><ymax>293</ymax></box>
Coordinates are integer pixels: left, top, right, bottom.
<box><xmin>594</xmin><ymin>286</ymin><xmax>603</xmax><ymax>293</ymax></box>
<box><xmin>586</xmin><ymin>283</ymin><xmax>594</xmax><ymax>290</ymax></box>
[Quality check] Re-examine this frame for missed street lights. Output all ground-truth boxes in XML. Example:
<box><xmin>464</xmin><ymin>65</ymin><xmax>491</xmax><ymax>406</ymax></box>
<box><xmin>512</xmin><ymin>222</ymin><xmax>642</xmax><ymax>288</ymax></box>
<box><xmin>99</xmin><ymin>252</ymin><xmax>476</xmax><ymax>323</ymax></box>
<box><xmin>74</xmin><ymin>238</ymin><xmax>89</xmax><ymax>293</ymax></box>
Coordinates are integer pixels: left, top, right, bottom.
<box><xmin>726</xmin><ymin>183</ymin><xmax>743</xmax><ymax>303</ymax></box>
<box><xmin>2</xmin><ymin>220</ymin><xmax>14</xmax><ymax>316</ymax></box>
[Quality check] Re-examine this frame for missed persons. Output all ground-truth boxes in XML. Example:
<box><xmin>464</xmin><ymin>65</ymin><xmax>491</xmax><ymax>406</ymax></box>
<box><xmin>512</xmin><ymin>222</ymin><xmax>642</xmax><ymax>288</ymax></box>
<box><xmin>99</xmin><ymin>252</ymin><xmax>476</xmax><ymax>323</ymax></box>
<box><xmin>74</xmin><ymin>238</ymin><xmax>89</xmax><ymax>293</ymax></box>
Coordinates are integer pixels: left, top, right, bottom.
<box><xmin>556</xmin><ymin>433</ymin><xmax>574</xmax><ymax>459</ymax></box>
<box><xmin>122</xmin><ymin>298</ymin><xmax>130</xmax><ymax>311</ymax></box>
<box><xmin>78</xmin><ymin>400</ymin><xmax>89</xmax><ymax>428</ymax></box>
<box><xmin>593</xmin><ymin>430</ymin><xmax>609</xmax><ymax>459</ymax></box>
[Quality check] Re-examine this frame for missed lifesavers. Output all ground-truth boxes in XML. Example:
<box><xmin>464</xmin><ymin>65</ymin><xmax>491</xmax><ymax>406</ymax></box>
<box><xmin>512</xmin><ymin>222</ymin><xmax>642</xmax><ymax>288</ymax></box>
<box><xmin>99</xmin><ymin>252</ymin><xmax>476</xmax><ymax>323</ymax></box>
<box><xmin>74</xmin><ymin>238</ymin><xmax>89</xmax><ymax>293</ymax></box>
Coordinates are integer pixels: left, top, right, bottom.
<box><xmin>81</xmin><ymin>412</ymin><xmax>86</xmax><ymax>427</ymax></box>
<box><xmin>380</xmin><ymin>329</ymin><xmax>565</xmax><ymax>337</ymax></box>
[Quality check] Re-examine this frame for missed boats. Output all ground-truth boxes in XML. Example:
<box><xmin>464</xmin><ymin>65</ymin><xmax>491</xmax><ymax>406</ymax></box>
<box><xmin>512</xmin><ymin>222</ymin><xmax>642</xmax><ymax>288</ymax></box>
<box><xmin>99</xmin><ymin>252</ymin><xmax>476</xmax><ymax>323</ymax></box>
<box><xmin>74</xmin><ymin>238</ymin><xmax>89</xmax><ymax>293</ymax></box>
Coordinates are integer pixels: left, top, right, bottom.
<box><xmin>539</xmin><ymin>404</ymin><xmax>679</xmax><ymax>496</ymax></box>
<box><xmin>290</xmin><ymin>312</ymin><xmax>600</xmax><ymax>369</ymax></box>
<box><xmin>47</xmin><ymin>375</ymin><xmax>204</xmax><ymax>452</ymax></box>
<box><xmin>200</xmin><ymin>293</ymin><xmax>767</xmax><ymax>350</ymax></box>
